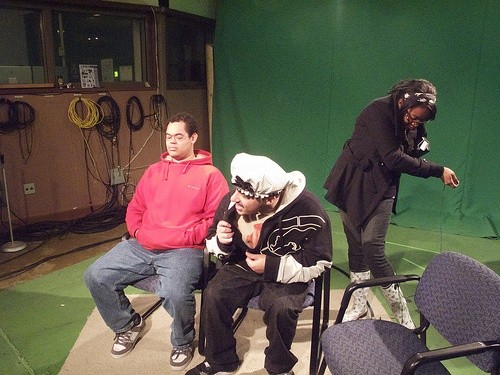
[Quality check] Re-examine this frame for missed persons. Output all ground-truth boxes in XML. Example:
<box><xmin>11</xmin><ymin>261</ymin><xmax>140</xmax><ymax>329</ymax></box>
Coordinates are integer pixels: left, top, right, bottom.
<box><xmin>324</xmin><ymin>79</ymin><xmax>460</xmax><ymax>337</ymax></box>
<box><xmin>84</xmin><ymin>112</ymin><xmax>230</xmax><ymax>371</ymax></box>
<box><xmin>185</xmin><ymin>152</ymin><xmax>332</xmax><ymax>375</ymax></box>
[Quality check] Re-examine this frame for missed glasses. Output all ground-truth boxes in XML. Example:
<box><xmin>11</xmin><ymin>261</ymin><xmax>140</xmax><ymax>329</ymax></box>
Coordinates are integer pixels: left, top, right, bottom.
<box><xmin>405</xmin><ymin>112</ymin><xmax>431</xmax><ymax>125</ymax></box>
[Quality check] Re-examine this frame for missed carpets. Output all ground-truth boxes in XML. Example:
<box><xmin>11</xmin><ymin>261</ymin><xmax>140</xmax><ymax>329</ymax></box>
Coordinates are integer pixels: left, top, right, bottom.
<box><xmin>57</xmin><ymin>287</ymin><xmax>392</xmax><ymax>375</ymax></box>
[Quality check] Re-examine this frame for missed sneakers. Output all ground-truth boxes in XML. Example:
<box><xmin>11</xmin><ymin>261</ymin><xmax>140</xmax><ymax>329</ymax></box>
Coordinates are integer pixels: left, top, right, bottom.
<box><xmin>185</xmin><ymin>359</ymin><xmax>240</xmax><ymax>375</ymax></box>
<box><xmin>169</xmin><ymin>345</ymin><xmax>194</xmax><ymax>370</ymax></box>
<box><xmin>110</xmin><ymin>312</ymin><xmax>146</xmax><ymax>358</ymax></box>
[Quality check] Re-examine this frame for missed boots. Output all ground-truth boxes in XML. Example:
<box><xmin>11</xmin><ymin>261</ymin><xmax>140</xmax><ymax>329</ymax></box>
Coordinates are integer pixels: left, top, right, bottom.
<box><xmin>379</xmin><ymin>272</ymin><xmax>416</xmax><ymax>330</ymax></box>
<box><xmin>342</xmin><ymin>270</ymin><xmax>371</xmax><ymax>323</ymax></box>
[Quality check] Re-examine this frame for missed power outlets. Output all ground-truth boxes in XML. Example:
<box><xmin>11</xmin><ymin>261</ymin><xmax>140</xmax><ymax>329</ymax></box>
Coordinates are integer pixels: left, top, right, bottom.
<box><xmin>22</xmin><ymin>182</ymin><xmax>36</xmax><ymax>195</ymax></box>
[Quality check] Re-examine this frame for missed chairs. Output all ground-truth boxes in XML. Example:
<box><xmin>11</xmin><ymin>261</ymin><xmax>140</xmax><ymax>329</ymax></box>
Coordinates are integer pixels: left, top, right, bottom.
<box><xmin>120</xmin><ymin>231</ymin><xmax>500</xmax><ymax>375</ymax></box>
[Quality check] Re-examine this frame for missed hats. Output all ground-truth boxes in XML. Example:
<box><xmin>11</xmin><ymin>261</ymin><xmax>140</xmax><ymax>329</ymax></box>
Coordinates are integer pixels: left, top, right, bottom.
<box><xmin>230</xmin><ymin>153</ymin><xmax>289</xmax><ymax>198</ymax></box>
